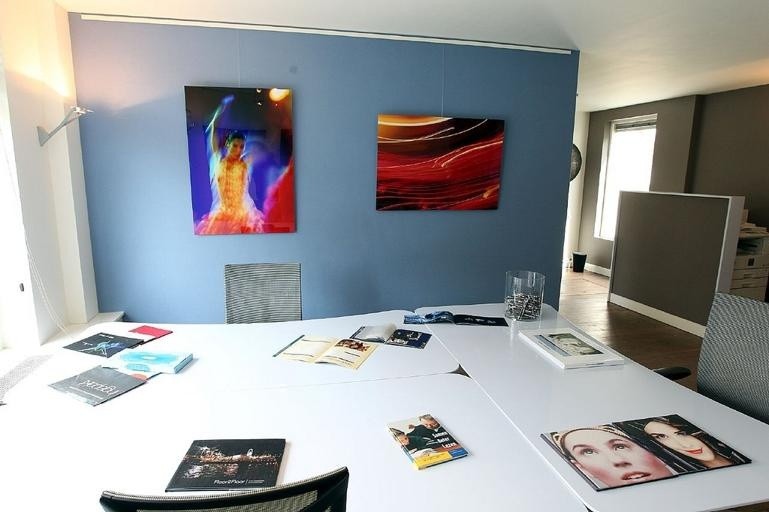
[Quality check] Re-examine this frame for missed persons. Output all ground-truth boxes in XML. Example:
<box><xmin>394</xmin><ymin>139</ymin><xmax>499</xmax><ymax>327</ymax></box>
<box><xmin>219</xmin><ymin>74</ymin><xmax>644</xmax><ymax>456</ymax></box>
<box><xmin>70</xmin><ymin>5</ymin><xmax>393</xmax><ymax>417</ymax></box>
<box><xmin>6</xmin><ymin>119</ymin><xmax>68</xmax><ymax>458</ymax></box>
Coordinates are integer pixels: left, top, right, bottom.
<box><xmin>636</xmin><ymin>417</ymin><xmax>749</xmax><ymax>471</ymax></box>
<box><xmin>390</xmin><ymin>424</ymin><xmax>434</xmax><ymax>446</ymax></box>
<box><xmin>195</xmin><ymin>94</ymin><xmax>270</xmax><ymax>233</ymax></box>
<box><xmin>411</xmin><ymin>413</ymin><xmax>453</xmax><ymax>444</ymax></box>
<box><xmin>554</xmin><ymin>425</ymin><xmax>676</xmax><ymax>486</ymax></box>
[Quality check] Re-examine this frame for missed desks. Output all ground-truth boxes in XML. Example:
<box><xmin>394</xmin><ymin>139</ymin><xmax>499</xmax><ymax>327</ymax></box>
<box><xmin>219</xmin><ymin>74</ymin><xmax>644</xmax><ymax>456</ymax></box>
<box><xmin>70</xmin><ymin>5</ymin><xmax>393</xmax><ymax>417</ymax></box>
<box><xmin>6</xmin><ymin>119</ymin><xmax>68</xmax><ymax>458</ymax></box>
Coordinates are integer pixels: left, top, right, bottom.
<box><xmin>0</xmin><ymin>299</ymin><xmax>769</xmax><ymax>512</ymax></box>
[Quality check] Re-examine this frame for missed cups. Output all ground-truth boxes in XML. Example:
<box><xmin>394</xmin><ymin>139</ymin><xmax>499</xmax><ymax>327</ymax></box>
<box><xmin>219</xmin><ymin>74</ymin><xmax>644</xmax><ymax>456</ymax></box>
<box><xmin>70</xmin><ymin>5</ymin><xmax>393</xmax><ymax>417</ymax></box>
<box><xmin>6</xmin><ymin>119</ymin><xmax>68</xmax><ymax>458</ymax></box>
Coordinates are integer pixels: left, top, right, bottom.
<box><xmin>505</xmin><ymin>269</ymin><xmax>546</xmax><ymax>321</ymax></box>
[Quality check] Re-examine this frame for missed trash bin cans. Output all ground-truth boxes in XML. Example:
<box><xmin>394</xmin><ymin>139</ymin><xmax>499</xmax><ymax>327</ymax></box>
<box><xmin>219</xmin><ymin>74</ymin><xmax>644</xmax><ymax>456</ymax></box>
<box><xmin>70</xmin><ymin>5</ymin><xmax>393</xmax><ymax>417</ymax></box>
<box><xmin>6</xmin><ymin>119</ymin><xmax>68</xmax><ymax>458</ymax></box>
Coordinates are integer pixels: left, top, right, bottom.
<box><xmin>573</xmin><ymin>252</ymin><xmax>587</xmax><ymax>273</ymax></box>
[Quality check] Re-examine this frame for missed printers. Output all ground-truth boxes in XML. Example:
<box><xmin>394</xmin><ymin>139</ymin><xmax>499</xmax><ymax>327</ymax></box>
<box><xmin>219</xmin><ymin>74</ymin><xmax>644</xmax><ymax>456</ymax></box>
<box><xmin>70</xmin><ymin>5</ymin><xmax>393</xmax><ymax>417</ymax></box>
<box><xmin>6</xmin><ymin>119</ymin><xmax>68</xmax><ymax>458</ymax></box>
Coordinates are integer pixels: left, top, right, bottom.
<box><xmin>730</xmin><ymin>209</ymin><xmax>769</xmax><ymax>300</ymax></box>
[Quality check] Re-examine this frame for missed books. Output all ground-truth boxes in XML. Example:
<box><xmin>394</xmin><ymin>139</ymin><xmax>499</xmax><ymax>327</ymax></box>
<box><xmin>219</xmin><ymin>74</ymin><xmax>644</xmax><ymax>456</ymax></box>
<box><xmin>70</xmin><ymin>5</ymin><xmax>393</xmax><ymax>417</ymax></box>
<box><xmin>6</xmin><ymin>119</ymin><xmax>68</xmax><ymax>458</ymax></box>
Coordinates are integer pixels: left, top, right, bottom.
<box><xmin>403</xmin><ymin>312</ymin><xmax>508</xmax><ymax>330</ymax></box>
<box><xmin>516</xmin><ymin>325</ymin><xmax>625</xmax><ymax>369</ymax></box>
<box><xmin>388</xmin><ymin>412</ymin><xmax>468</xmax><ymax>471</ymax></box>
<box><xmin>271</xmin><ymin>333</ymin><xmax>379</xmax><ymax>371</ymax></box>
<box><xmin>42</xmin><ymin>323</ymin><xmax>193</xmax><ymax>407</ymax></box>
<box><xmin>540</xmin><ymin>415</ymin><xmax>751</xmax><ymax>490</ymax></box>
<box><xmin>349</xmin><ymin>316</ymin><xmax>434</xmax><ymax>350</ymax></box>
<box><xmin>164</xmin><ymin>435</ymin><xmax>286</xmax><ymax>495</ymax></box>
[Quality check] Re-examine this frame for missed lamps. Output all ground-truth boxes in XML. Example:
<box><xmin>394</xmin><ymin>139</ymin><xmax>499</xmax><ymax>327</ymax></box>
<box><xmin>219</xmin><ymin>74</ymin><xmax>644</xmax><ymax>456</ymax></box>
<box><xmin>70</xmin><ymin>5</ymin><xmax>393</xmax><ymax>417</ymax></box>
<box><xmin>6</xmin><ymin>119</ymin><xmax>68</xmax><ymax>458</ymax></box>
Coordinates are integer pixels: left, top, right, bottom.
<box><xmin>36</xmin><ymin>104</ymin><xmax>93</xmax><ymax>148</ymax></box>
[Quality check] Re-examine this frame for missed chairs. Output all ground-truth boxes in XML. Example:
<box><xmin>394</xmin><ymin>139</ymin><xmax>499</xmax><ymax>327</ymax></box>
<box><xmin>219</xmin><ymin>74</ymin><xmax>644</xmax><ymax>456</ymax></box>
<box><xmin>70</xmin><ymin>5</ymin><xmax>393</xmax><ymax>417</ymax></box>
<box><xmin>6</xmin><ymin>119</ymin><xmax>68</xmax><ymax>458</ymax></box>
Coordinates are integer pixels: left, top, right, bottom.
<box><xmin>223</xmin><ymin>261</ymin><xmax>304</xmax><ymax>323</ymax></box>
<box><xmin>650</xmin><ymin>292</ymin><xmax>769</xmax><ymax>425</ymax></box>
<box><xmin>97</xmin><ymin>466</ymin><xmax>350</xmax><ymax>512</ymax></box>
